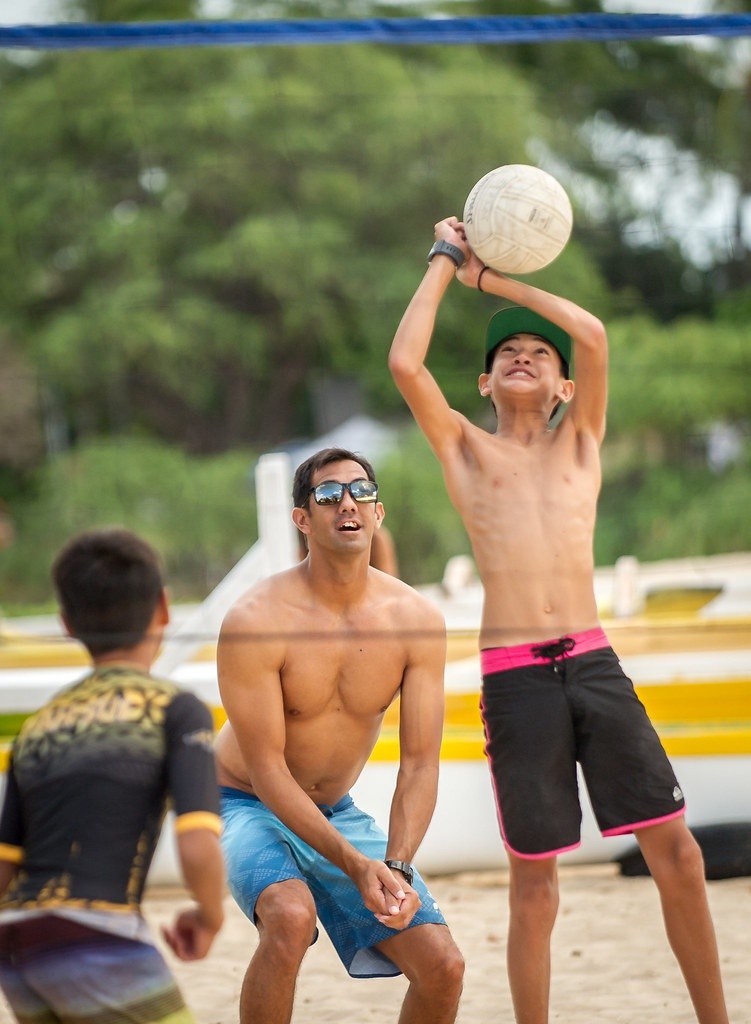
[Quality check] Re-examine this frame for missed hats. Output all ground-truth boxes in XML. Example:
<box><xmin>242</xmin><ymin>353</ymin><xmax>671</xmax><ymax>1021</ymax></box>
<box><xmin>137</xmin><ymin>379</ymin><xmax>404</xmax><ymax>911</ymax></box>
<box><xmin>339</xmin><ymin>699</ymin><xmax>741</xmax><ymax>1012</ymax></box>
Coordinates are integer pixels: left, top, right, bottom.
<box><xmin>484</xmin><ymin>308</ymin><xmax>571</xmax><ymax>423</ymax></box>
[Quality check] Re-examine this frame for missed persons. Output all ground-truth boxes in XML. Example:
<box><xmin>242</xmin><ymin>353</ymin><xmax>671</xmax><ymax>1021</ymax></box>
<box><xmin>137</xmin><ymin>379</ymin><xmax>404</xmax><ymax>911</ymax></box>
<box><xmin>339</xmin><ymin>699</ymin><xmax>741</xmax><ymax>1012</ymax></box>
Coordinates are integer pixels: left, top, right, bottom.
<box><xmin>214</xmin><ymin>445</ymin><xmax>464</xmax><ymax>1024</ymax></box>
<box><xmin>388</xmin><ymin>214</ymin><xmax>728</xmax><ymax>1024</ymax></box>
<box><xmin>0</xmin><ymin>530</ymin><xmax>224</xmax><ymax>1024</ymax></box>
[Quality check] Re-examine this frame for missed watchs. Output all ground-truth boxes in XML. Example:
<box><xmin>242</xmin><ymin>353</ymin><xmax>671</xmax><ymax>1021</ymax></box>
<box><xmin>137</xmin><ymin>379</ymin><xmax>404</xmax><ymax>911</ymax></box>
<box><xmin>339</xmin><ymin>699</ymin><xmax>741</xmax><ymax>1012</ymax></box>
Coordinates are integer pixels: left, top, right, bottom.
<box><xmin>427</xmin><ymin>238</ymin><xmax>465</xmax><ymax>270</ymax></box>
<box><xmin>385</xmin><ymin>859</ymin><xmax>413</xmax><ymax>886</ymax></box>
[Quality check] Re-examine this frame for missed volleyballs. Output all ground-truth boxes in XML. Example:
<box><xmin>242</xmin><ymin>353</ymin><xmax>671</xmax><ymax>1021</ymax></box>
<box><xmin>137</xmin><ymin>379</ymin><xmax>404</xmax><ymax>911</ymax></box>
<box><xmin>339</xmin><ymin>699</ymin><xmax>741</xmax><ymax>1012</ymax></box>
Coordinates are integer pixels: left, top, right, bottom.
<box><xmin>461</xmin><ymin>162</ymin><xmax>575</xmax><ymax>276</ymax></box>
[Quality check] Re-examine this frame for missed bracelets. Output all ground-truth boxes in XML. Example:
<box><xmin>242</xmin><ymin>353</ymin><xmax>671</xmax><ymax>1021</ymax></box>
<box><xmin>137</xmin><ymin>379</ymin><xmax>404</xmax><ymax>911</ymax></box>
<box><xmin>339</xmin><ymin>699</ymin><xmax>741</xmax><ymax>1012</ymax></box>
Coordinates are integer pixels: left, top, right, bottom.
<box><xmin>478</xmin><ymin>266</ymin><xmax>490</xmax><ymax>292</ymax></box>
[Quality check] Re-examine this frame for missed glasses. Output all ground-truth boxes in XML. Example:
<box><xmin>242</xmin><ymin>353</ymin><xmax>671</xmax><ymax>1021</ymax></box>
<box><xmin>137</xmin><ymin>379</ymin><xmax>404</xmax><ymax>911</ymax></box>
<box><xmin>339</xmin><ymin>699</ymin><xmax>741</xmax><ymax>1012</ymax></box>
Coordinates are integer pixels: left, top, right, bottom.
<box><xmin>301</xmin><ymin>479</ymin><xmax>379</xmax><ymax>505</ymax></box>
